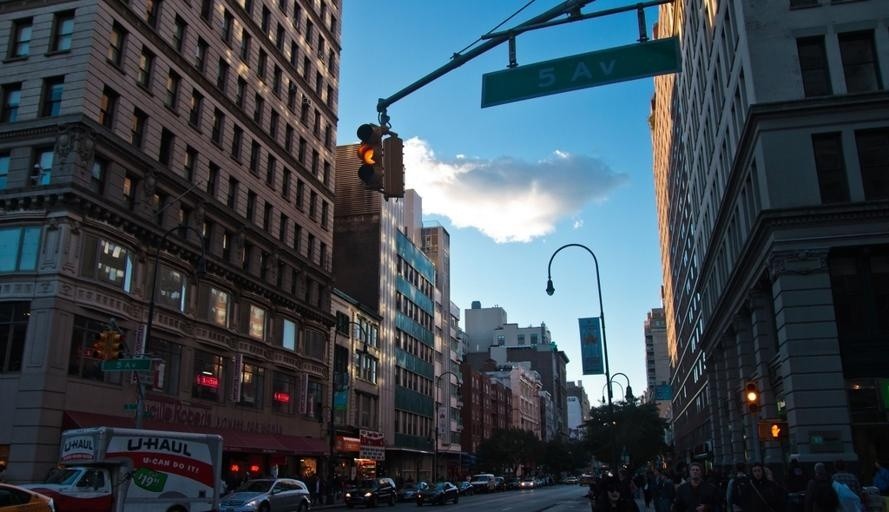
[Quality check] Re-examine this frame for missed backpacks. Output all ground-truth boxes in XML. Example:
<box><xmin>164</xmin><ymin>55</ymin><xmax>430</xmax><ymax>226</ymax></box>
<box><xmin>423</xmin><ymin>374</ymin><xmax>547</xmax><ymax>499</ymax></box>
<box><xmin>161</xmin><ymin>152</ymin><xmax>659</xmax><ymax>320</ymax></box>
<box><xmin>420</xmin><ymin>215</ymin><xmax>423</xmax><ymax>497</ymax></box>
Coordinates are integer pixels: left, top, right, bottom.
<box><xmin>732</xmin><ymin>477</ymin><xmax>749</xmax><ymax>506</ymax></box>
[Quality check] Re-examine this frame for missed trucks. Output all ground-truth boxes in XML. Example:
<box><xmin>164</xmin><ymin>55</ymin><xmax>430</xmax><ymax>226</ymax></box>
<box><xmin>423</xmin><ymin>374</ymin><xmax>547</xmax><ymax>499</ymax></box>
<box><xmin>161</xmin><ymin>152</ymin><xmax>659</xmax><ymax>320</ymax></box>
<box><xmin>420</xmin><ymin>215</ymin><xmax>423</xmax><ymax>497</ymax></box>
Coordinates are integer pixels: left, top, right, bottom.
<box><xmin>17</xmin><ymin>426</ymin><xmax>224</xmax><ymax>512</ymax></box>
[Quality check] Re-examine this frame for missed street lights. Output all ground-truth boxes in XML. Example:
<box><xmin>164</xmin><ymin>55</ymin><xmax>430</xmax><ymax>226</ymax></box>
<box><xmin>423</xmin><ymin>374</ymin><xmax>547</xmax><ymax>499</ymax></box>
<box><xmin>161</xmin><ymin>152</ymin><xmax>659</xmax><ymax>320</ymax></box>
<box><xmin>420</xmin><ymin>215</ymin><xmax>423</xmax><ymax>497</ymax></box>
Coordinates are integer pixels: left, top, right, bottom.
<box><xmin>136</xmin><ymin>225</ymin><xmax>207</xmax><ymax>429</ymax></box>
<box><xmin>329</xmin><ymin>321</ymin><xmax>369</xmax><ymax>504</ymax></box>
<box><xmin>436</xmin><ymin>371</ymin><xmax>463</xmax><ymax>482</ymax></box>
<box><xmin>602</xmin><ymin>372</ymin><xmax>634</xmax><ymax>404</ymax></box>
<box><xmin>546</xmin><ymin>243</ymin><xmax>635</xmax><ymax>451</ymax></box>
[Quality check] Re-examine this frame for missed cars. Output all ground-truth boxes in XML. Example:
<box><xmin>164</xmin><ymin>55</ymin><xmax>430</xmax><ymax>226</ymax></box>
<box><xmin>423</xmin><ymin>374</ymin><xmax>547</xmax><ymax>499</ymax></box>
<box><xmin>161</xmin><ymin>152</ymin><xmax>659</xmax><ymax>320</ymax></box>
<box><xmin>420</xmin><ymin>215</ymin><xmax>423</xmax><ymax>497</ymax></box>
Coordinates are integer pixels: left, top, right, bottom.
<box><xmin>567</xmin><ymin>472</ymin><xmax>595</xmax><ymax>487</ymax></box>
<box><xmin>396</xmin><ymin>473</ymin><xmax>507</xmax><ymax>508</ymax></box>
<box><xmin>0</xmin><ymin>483</ymin><xmax>55</xmax><ymax>512</ymax></box>
<box><xmin>344</xmin><ymin>478</ymin><xmax>396</xmax><ymax>507</ymax></box>
<box><xmin>508</xmin><ymin>473</ymin><xmax>550</xmax><ymax>490</ymax></box>
<box><xmin>218</xmin><ymin>478</ymin><xmax>313</xmax><ymax>512</ymax></box>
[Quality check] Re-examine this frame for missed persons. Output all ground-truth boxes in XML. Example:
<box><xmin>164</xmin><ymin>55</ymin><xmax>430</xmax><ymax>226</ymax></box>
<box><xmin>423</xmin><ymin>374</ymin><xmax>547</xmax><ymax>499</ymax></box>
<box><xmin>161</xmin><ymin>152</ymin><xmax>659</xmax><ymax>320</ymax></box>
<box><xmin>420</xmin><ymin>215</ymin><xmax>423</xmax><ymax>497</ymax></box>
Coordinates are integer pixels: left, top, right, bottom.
<box><xmin>219</xmin><ymin>469</ymin><xmax>452</xmax><ymax>506</ymax></box>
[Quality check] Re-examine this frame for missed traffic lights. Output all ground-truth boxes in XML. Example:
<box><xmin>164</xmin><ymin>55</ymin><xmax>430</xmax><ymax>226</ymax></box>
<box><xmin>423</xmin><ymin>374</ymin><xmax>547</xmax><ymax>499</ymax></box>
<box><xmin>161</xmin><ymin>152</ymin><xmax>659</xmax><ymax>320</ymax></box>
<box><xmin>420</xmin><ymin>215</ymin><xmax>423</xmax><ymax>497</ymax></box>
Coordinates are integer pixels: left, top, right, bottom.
<box><xmin>90</xmin><ymin>330</ymin><xmax>126</xmax><ymax>363</ymax></box>
<box><xmin>357</xmin><ymin>123</ymin><xmax>385</xmax><ymax>190</ymax></box>
<box><xmin>745</xmin><ymin>381</ymin><xmax>760</xmax><ymax>415</ymax></box>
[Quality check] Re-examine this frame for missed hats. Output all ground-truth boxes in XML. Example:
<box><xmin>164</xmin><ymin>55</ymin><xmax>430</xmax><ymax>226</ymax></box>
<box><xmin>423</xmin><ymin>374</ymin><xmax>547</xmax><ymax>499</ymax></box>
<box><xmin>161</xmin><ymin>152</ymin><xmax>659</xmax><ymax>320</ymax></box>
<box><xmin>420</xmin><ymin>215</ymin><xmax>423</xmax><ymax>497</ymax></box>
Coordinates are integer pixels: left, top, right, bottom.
<box><xmin>815</xmin><ymin>463</ymin><xmax>824</xmax><ymax>473</ymax></box>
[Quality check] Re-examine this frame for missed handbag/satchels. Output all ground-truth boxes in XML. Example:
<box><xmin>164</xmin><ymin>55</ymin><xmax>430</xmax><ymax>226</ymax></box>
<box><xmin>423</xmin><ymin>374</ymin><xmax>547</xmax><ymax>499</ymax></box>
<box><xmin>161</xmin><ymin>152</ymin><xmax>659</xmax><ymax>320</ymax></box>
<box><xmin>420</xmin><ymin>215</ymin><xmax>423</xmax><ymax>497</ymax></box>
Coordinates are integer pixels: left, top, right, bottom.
<box><xmin>832</xmin><ymin>481</ymin><xmax>863</xmax><ymax>512</ymax></box>
